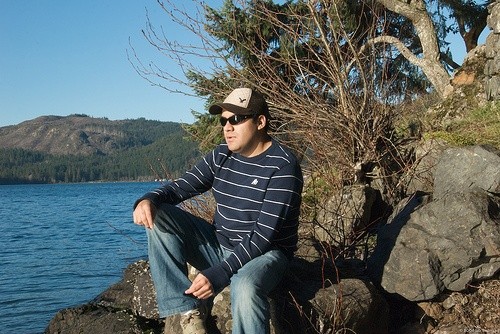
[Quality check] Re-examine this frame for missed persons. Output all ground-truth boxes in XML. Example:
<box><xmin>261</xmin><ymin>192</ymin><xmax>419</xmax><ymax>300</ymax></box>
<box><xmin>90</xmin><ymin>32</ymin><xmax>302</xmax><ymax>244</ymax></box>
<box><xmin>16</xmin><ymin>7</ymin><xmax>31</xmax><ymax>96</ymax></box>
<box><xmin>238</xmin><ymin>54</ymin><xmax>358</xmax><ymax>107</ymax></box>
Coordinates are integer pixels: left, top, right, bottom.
<box><xmin>133</xmin><ymin>88</ymin><xmax>304</xmax><ymax>334</ymax></box>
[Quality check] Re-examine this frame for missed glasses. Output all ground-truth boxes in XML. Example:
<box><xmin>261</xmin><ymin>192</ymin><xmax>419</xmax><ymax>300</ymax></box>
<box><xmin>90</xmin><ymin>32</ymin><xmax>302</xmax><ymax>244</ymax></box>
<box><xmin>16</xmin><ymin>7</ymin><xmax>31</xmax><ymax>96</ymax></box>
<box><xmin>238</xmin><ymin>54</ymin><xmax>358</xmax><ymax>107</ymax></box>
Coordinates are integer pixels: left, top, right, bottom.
<box><xmin>219</xmin><ymin>115</ymin><xmax>253</xmax><ymax>126</ymax></box>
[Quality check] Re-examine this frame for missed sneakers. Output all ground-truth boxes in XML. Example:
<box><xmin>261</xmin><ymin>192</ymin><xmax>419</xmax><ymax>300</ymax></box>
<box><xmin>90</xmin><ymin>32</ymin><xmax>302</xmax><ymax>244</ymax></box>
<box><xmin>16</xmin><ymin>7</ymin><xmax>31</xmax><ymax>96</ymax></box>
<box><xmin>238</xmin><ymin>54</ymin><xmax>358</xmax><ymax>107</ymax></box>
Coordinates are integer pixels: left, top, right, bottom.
<box><xmin>180</xmin><ymin>307</ymin><xmax>209</xmax><ymax>334</ymax></box>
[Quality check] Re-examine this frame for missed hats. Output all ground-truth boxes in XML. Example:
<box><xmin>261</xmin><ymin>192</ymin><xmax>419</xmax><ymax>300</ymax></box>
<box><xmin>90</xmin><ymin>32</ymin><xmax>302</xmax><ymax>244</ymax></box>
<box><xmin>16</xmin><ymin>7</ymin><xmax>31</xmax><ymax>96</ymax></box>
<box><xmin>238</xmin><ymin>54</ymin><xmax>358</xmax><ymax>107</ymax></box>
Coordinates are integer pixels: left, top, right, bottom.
<box><xmin>209</xmin><ymin>88</ymin><xmax>268</xmax><ymax>120</ymax></box>
